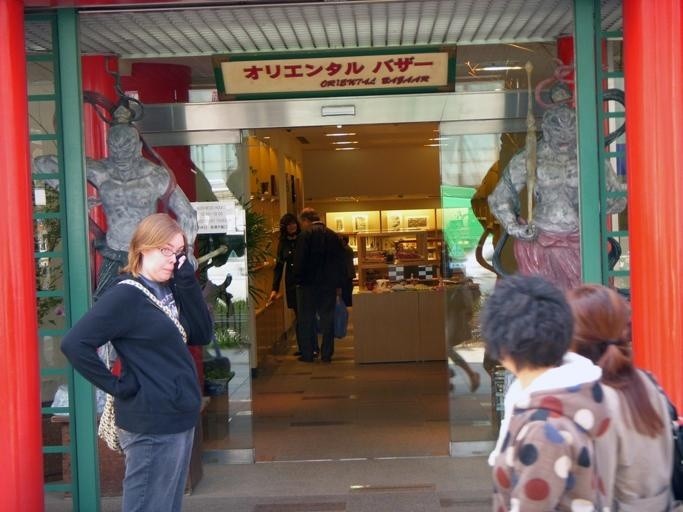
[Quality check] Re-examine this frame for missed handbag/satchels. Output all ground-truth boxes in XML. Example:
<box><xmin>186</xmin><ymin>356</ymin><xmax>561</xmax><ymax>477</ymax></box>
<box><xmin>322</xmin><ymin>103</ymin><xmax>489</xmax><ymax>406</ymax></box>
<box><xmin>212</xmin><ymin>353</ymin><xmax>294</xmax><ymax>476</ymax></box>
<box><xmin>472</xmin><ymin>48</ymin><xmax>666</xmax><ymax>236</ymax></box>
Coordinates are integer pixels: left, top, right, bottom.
<box><xmin>316</xmin><ymin>303</ymin><xmax>348</xmax><ymax>339</ymax></box>
<box><xmin>98</xmin><ymin>392</ymin><xmax>121</xmax><ymax>452</ymax></box>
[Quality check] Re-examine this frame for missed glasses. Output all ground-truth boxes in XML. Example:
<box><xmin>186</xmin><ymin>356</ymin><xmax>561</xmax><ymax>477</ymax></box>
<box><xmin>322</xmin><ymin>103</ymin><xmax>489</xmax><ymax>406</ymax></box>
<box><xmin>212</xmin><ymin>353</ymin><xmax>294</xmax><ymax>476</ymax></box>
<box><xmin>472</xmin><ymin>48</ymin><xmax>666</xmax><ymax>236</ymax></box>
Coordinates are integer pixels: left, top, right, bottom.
<box><xmin>158</xmin><ymin>247</ymin><xmax>183</xmax><ymax>257</ymax></box>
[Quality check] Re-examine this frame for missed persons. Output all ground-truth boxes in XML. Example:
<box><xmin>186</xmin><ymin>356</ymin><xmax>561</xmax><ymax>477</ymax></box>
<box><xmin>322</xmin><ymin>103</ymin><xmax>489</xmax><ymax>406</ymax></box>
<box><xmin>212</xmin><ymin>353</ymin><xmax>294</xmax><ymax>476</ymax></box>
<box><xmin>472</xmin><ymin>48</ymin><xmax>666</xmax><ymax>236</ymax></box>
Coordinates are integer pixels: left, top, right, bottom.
<box><xmin>486</xmin><ymin>100</ymin><xmax>629</xmax><ymax>291</ymax></box>
<box><xmin>479</xmin><ymin>273</ymin><xmax>618</xmax><ymax>512</ymax></box>
<box><xmin>265</xmin><ymin>213</ymin><xmax>320</xmax><ymax>357</ymax></box>
<box><xmin>61</xmin><ymin>212</ymin><xmax>212</xmax><ymax>512</ymax></box>
<box><xmin>33</xmin><ymin>121</ymin><xmax>200</xmax><ymax>413</ymax></box>
<box><xmin>338</xmin><ymin>234</ymin><xmax>356</xmax><ymax>307</ymax></box>
<box><xmin>566</xmin><ymin>283</ymin><xmax>682</xmax><ymax>512</ymax></box>
<box><xmin>290</xmin><ymin>206</ymin><xmax>345</xmax><ymax>364</ymax></box>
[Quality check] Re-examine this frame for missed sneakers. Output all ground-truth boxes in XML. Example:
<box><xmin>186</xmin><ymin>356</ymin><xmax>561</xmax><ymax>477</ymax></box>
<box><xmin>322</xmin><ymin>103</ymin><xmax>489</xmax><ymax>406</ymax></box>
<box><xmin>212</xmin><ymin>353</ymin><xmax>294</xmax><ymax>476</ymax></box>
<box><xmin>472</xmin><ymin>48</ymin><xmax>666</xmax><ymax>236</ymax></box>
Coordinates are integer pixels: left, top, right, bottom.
<box><xmin>469</xmin><ymin>373</ymin><xmax>481</xmax><ymax>393</ymax></box>
<box><xmin>293</xmin><ymin>351</ymin><xmax>332</xmax><ymax>362</ymax></box>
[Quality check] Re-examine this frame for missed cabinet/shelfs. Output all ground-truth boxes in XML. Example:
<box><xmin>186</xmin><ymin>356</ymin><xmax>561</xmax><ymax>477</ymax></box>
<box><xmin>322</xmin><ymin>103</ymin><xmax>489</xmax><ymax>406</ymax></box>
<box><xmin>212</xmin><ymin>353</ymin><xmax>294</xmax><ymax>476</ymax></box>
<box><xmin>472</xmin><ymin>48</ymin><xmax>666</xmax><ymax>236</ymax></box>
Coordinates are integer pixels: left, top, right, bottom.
<box><xmin>325</xmin><ymin>209</ymin><xmax>474</xmax><ymax>364</ymax></box>
<box><xmin>248</xmin><ymin>137</ymin><xmax>305</xmax><ymax>378</ymax></box>
